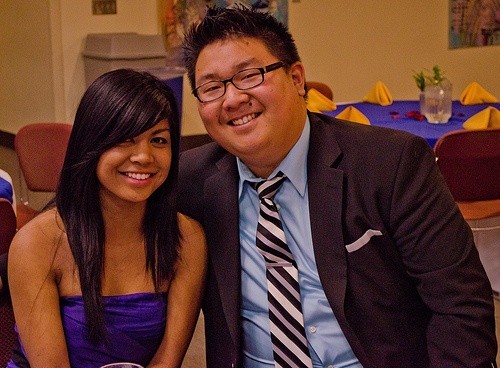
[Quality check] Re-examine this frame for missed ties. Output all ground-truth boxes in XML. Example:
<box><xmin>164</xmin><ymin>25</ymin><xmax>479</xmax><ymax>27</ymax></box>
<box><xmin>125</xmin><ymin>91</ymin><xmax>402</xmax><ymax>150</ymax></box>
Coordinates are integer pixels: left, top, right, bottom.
<box><xmin>245</xmin><ymin>170</ymin><xmax>313</xmax><ymax>368</ymax></box>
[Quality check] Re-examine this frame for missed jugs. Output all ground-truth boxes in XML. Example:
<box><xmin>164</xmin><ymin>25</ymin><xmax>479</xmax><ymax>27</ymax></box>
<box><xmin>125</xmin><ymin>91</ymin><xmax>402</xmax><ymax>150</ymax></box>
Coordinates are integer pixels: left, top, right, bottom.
<box><xmin>424</xmin><ymin>76</ymin><xmax>452</xmax><ymax>124</ymax></box>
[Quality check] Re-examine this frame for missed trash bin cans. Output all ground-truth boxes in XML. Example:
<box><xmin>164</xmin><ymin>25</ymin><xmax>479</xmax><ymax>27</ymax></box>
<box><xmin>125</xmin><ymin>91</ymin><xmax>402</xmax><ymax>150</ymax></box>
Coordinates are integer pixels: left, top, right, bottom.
<box><xmin>83</xmin><ymin>31</ymin><xmax>187</xmax><ymax>137</ymax></box>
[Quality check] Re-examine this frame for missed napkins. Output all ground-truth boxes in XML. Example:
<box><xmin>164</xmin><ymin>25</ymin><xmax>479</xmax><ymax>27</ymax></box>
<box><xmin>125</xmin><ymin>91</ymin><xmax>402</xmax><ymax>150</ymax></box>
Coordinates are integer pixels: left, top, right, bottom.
<box><xmin>462</xmin><ymin>106</ymin><xmax>500</xmax><ymax>130</ymax></box>
<box><xmin>363</xmin><ymin>81</ymin><xmax>393</xmax><ymax>106</ymax></box>
<box><xmin>306</xmin><ymin>88</ymin><xmax>336</xmax><ymax>113</ymax></box>
<box><xmin>460</xmin><ymin>83</ymin><xmax>499</xmax><ymax>105</ymax></box>
<box><xmin>335</xmin><ymin>105</ymin><xmax>370</xmax><ymax>125</ymax></box>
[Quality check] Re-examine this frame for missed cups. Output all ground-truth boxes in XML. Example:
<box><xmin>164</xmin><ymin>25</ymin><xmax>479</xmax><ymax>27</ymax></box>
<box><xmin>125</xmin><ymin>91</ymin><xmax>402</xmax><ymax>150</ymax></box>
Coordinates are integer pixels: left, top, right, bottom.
<box><xmin>100</xmin><ymin>362</ymin><xmax>143</xmax><ymax>368</ymax></box>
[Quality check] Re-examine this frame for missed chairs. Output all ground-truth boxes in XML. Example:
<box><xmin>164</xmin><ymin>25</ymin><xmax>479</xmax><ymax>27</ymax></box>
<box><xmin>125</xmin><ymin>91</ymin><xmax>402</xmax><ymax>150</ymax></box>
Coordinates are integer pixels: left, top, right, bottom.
<box><xmin>306</xmin><ymin>81</ymin><xmax>333</xmax><ymax>101</ymax></box>
<box><xmin>433</xmin><ymin>129</ymin><xmax>500</xmax><ymax>231</ymax></box>
<box><xmin>0</xmin><ymin>122</ymin><xmax>72</xmax><ymax>257</ymax></box>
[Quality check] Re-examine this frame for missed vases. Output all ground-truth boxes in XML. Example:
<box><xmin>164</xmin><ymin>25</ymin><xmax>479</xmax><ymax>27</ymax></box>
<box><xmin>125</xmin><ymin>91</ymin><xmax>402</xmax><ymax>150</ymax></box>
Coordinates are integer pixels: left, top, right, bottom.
<box><xmin>421</xmin><ymin>83</ymin><xmax>451</xmax><ymax>124</ymax></box>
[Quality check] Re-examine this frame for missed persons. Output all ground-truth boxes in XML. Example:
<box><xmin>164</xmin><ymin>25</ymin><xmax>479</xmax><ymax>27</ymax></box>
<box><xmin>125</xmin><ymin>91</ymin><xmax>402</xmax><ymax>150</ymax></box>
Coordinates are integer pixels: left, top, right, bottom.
<box><xmin>8</xmin><ymin>68</ymin><xmax>206</xmax><ymax>368</ymax></box>
<box><xmin>179</xmin><ymin>3</ymin><xmax>497</xmax><ymax>368</ymax></box>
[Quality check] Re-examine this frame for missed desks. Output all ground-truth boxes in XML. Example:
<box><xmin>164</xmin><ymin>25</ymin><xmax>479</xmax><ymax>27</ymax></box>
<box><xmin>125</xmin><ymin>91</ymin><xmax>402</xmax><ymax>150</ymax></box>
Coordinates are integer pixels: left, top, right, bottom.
<box><xmin>321</xmin><ymin>100</ymin><xmax>500</xmax><ymax>149</ymax></box>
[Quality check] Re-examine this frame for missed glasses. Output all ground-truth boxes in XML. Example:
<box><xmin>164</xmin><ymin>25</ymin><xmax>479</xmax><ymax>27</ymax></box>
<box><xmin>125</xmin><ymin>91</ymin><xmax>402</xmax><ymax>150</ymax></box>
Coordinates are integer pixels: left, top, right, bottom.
<box><xmin>192</xmin><ymin>60</ymin><xmax>284</xmax><ymax>102</ymax></box>
